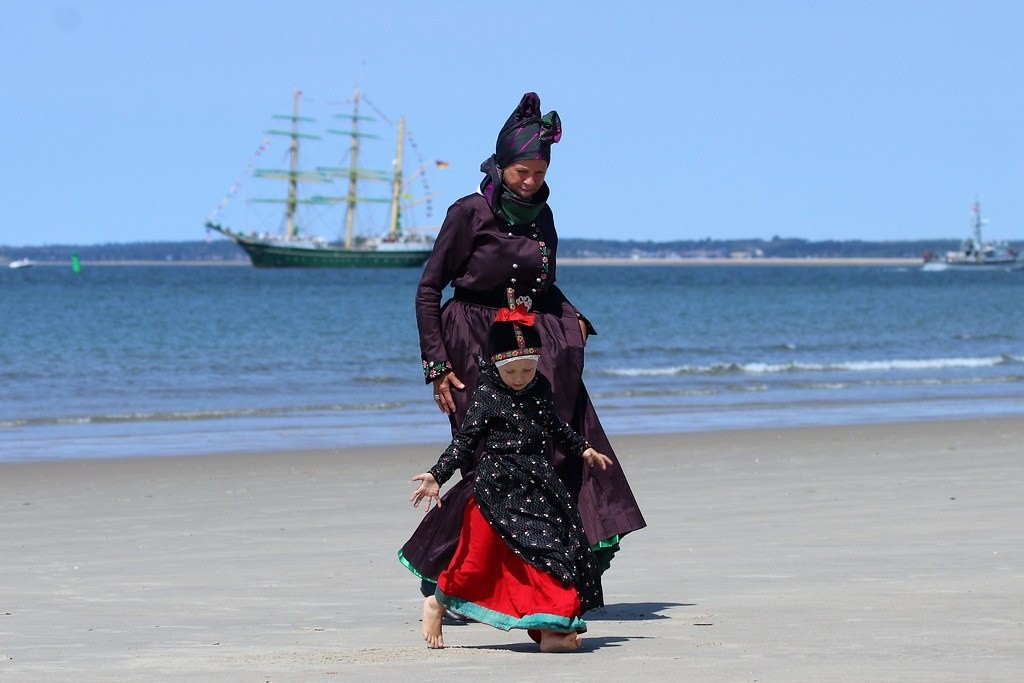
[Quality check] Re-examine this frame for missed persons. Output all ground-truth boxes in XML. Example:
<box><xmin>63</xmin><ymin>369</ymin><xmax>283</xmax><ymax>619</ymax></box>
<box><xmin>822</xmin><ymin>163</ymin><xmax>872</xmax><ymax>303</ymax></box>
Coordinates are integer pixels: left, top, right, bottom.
<box><xmin>412</xmin><ymin>286</ymin><xmax>612</xmax><ymax>654</ymax></box>
<box><xmin>416</xmin><ymin>94</ymin><xmax>647</xmax><ymax>625</ymax></box>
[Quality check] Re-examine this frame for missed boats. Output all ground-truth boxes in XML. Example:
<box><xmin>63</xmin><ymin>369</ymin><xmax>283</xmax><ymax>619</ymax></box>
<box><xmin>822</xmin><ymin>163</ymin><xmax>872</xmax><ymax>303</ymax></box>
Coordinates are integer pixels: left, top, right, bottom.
<box><xmin>921</xmin><ymin>191</ymin><xmax>1023</xmax><ymax>269</ymax></box>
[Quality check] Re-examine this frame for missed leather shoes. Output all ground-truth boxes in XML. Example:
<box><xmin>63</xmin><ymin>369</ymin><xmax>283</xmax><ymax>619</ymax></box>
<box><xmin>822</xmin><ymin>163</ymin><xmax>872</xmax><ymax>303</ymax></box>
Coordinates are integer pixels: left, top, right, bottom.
<box><xmin>439</xmin><ymin>608</ymin><xmax>467</xmax><ymax>626</ymax></box>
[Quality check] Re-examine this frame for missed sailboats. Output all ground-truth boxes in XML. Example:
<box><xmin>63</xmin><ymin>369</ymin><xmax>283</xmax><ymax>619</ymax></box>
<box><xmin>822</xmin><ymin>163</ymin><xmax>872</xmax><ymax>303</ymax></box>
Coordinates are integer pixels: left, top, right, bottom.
<box><xmin>203</xmin><ymin>78</ymin><xmax>453</xmax><ymax>268</ymax></box>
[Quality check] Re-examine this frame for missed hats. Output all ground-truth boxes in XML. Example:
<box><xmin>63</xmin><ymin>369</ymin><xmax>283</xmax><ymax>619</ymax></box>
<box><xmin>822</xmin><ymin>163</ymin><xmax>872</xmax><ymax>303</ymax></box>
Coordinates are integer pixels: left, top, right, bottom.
<box><xmin>485</xmin><ymin>302</ymin><xmax>542</xmax><ymax>367</ymax></box>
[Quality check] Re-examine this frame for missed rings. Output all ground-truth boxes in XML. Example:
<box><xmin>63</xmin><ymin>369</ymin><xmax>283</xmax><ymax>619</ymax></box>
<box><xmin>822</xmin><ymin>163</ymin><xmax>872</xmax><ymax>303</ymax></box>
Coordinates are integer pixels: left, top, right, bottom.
<box><xmin>434</xmin><ymin>394</ymin><xmax>439</xmax><ymax>400</ymax></box>
